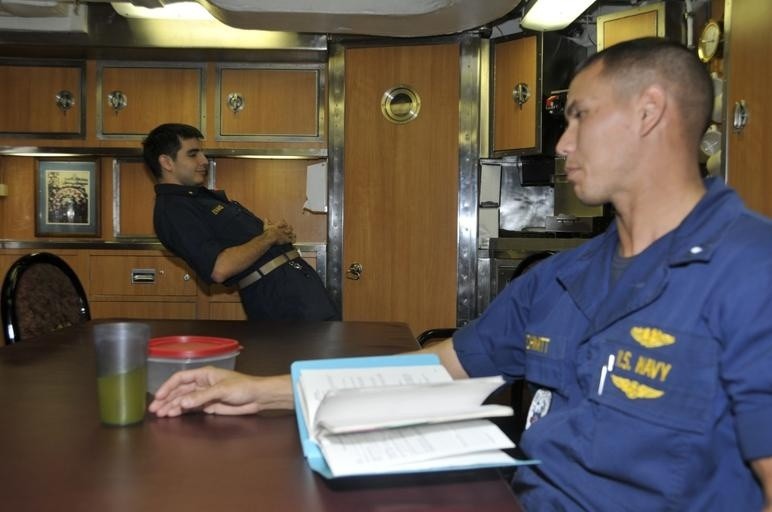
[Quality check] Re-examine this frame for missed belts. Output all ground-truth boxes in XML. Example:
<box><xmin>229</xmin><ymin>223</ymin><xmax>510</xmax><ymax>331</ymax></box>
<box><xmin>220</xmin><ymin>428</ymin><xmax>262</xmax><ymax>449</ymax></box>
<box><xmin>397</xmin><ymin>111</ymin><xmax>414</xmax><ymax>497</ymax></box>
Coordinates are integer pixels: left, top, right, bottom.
<box><xmin>231</xmin><ymin>248</ymin><xmax>301</xmax><ymax>293</ymax></box>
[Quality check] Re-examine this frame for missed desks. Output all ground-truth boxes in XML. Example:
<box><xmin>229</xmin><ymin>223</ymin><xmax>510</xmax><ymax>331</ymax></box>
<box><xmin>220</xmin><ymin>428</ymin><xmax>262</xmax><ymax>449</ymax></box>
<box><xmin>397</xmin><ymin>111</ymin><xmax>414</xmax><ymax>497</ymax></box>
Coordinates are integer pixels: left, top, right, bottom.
<box><xmin>0</xmin><ymin>317</ymin><xmax>530</xmax><ymax>512</ymax></box>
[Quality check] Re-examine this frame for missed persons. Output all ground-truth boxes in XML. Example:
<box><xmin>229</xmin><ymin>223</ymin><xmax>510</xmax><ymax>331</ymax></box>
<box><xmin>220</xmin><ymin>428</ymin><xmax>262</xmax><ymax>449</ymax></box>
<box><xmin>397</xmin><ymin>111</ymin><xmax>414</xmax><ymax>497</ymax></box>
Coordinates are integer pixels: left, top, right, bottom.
<box><xmin>141</xmin><ymin>123</ymin><xmax>337</xmax><ymax>322</ymax></box>
<box><xmin>148</xmin><ymin>36</ymin><xmax>772</xmax><ymax>512</ymax></box>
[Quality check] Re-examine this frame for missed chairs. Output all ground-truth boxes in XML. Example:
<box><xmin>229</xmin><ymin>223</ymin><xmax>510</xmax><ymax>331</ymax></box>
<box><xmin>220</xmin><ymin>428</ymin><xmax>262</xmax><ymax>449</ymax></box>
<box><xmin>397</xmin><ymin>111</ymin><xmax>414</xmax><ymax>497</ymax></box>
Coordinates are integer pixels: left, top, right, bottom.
<box><xmin>0</xmin><ymin>252</ymin><xmax>91</xmax><ymax>345</ymax></box>
<box><xmin>415</xmin><ymin>251</ymin><xmax>559</xmax><ymax>425</ymax></box>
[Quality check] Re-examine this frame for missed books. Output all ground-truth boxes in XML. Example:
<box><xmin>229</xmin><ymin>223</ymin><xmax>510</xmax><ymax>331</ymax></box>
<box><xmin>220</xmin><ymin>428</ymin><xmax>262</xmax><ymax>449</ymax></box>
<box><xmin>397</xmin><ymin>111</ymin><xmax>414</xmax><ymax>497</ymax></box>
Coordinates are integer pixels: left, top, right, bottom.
<box><xmin>290</xmin><ymin>353</ymin><xmax>543</xmax><ymax>479</ymax></box>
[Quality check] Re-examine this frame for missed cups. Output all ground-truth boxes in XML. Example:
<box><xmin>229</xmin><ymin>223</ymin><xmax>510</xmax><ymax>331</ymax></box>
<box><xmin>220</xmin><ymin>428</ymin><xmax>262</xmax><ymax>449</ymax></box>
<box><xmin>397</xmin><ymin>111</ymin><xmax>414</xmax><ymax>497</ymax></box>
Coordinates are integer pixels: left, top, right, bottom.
<box><xmin>700</xmin><ymin>69</ymin><xmax>723</xmax><ymax>175</ymax></box>
<box><xmin>93</xmin><ymin>321</ymin><xmax>150</xmax><ymax>427</ymax></box>
